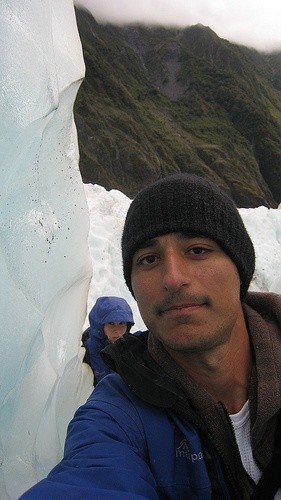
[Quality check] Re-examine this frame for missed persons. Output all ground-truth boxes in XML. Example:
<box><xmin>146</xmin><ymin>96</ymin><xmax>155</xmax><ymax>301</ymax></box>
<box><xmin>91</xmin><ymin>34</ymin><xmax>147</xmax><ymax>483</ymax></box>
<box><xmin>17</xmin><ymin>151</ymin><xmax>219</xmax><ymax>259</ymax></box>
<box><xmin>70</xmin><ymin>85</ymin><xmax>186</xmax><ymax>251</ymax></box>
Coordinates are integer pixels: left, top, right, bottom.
<box><xmin>80</xmin><ymin>295</ymin><xmax>135</xmax><ymax>380</ymax></box>
<box><xmin>13</xmin><ymin>173</ymin><xmax>281</xmax><ymax>500</ymax></box>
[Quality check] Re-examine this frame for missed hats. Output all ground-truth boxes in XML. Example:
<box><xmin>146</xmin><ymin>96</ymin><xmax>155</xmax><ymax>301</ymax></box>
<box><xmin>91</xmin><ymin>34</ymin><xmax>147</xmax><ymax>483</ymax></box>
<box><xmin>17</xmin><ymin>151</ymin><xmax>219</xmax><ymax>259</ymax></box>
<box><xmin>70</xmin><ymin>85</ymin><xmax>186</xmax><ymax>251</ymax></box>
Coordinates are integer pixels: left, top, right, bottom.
<box><xmin>121</xmin><ymin>172</ymin><xmax>256</xmax><ymax>303</ymax></box>
